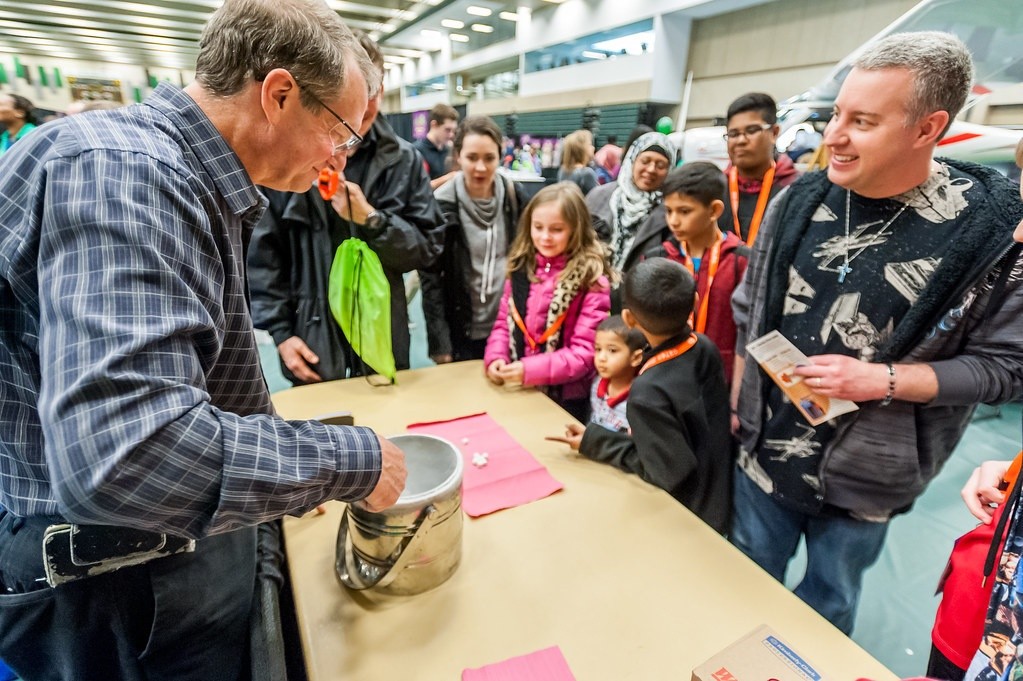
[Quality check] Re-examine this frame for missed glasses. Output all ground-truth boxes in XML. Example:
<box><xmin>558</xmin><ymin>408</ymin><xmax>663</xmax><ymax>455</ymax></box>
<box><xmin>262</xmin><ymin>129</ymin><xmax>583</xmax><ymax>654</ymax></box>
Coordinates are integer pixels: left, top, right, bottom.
<box><xmin>723</xmin><ymin>125</ymin><xmax>772</xmax><ymax>143</ymax></box>
<box><xmin>255</xmin><ymin>75</ymin><xmax>363</xmax><ymax>149</ymax></box>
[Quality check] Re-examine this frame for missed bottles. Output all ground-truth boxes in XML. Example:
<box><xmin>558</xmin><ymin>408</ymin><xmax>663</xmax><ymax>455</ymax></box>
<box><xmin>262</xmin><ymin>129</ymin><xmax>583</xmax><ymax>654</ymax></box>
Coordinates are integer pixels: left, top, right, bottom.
<box><xmin>589</xmin><ymin>161</ymin><xmax>611</xmax><ymax>184</ymax></box>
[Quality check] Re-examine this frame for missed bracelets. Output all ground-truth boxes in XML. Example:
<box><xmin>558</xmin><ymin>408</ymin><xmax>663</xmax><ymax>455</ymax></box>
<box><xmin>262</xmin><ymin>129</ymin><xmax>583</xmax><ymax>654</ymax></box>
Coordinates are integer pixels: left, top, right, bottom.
<box><xmin>880</xmin><ymin>363</ymin><xmax>897</xmax><ymax>410</ymax></box>
<box><xmin>730</xmin><ymin>408</ymin><xmax>738</xmax><ymax>414</ymax></box>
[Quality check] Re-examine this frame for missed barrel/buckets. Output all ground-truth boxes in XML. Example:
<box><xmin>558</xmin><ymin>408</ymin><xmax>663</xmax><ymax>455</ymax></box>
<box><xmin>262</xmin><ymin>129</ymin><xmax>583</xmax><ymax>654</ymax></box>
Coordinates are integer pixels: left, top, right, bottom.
<box><xmin>335</xmin><ymin>434</ymin><xmax>463</xmax><ymax>595</ymax></box>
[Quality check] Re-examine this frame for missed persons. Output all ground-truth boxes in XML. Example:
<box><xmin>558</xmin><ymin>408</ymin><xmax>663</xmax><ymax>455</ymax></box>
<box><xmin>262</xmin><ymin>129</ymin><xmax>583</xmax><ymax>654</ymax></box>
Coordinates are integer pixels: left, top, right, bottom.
<box><xmin>543</xmin><ymin>255</ymin><xmax>734</xmax><ymax>543</ymax></box>
<box><xmin>716</xmin><ymin>94</ymin><xmax>802</xmax><ymax>249</ymax></box>
<box><xmin>0</xmin><ymin>92</ymin><xmax>38</xmax><ymax>156</ymax></box>
<box><xmin>480</xmin><ymin>180</ymin><xmax>612</xmax><ymax>428</ymax></box>
<box><xmin>730</xmin><ymin>34</ymin><xmax>1022</xmax><ymax>638</ymax></box>
<box><xmin>500</xmin><ymin>126</ymin><xmax>651</xmax><ymax>196</ymax></box>
<box><xmin>924</xmin><ymin>144</ymin><xmax>1023</xmax><ymax>680</ymax></box>
<box><xmin>231</xmin><ymin>32</ymin><xmax>443</xmax><ymax>382</ymax></box>
<box><xmin>585</xmin><ymin>129</ymin><xmax>677</xmax><ymax>294</ymax></box>
<box><xmin>587</xmin><ymin>314</ymin><xmax>648</xmax><ymax>436</ymax></box>
<box><xmin>409</xmin><ymin>101</ymin><xmax>460</xmax><ymax>176</ymax></box>
<box><xmin>419</xmin><ymin>115</ymin><xmax>531</xmax><ymax>363</ymax></box>
<box><xmin>639</xmin><ymin>161</ymin><xmax>753</xmax><ymax>438</ymax></box>
<box><xmin>0</xmin><ymin>0</ymin><xmax>407</xmax><ymax>681</ymax></box>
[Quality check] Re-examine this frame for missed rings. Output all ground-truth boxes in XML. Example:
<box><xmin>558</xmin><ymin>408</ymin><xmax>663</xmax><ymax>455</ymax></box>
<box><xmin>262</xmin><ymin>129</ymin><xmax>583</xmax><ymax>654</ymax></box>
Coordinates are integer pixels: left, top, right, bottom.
<box><xmin>816</xmin><ymin>377</ymin><xmax>821</xmax><ymax>389</ymax></box>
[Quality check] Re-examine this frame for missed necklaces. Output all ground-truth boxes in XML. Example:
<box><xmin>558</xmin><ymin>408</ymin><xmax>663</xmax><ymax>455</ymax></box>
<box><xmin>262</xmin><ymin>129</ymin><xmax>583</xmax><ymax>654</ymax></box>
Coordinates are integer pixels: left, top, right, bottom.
<box><xmin>835</xmin><ymin>176</ymin><xmax>923</xmax><ymax>284</ymax></box>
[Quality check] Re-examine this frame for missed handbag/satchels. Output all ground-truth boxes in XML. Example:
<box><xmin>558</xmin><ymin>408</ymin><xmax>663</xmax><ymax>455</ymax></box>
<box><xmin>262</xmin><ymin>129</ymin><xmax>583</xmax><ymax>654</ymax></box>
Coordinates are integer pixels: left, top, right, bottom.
<box><xmin>928</xmin><ymin>508</ymin><xmax>1014</xmax><ymax>678</ymax></box>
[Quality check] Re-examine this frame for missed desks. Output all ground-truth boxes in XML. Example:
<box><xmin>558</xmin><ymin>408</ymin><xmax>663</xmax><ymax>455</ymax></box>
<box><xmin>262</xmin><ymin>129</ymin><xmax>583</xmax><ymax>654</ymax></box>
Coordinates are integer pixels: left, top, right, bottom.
<box><xmin>267</xmin><ymin>359</ymin><xmax>902</xmax><ymax>681</ymax></box>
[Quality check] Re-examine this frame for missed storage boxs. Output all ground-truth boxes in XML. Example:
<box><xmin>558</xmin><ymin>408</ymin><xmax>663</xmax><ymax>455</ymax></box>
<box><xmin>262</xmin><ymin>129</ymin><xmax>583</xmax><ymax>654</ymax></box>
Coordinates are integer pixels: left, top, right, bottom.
<box><xmin>690</xmin><ymin>623</ymin><xmax>828</xmax><ymax>681</ymax></box>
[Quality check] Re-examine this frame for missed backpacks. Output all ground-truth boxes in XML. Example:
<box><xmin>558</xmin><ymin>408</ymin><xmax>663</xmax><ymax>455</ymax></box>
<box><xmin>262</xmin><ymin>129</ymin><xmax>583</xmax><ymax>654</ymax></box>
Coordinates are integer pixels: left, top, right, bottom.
<box><xmin>327</xmin><ymin>185</ymin><xmax>396</xmax><ymax>386</ymax></box>
